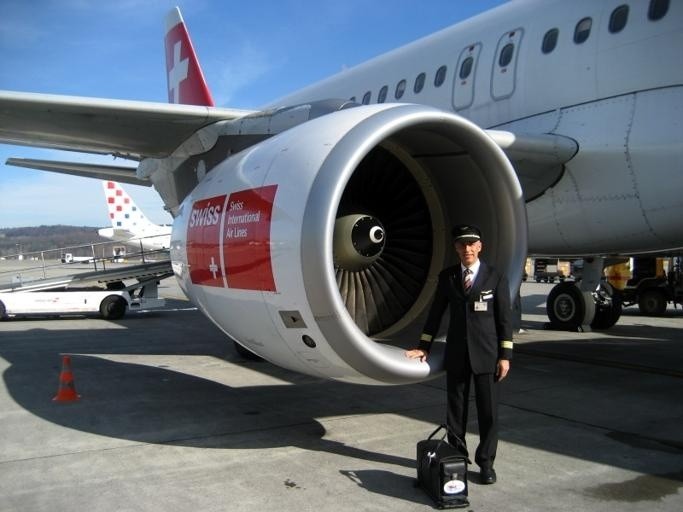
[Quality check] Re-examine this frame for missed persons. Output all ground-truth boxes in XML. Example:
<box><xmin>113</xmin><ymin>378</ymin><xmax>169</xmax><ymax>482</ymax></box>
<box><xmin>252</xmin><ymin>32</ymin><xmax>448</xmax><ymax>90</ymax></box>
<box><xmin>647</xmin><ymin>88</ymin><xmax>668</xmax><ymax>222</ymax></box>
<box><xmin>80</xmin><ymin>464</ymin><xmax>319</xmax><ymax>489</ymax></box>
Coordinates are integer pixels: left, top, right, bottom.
<box><xmin>404</xmin><ymin>224</ymin><xmax>516</xmax><ymax>485</ymax></box>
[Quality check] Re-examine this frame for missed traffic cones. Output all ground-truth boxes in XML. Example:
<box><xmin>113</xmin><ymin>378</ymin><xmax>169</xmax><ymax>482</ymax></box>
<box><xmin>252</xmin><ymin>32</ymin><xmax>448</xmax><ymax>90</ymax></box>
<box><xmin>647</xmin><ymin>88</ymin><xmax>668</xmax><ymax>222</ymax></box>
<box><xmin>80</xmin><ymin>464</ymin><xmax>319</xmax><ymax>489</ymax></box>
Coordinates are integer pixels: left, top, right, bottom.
<box><xmin>52</xmin><ymin>356</ymin><xmax>83</xmax><ymax>402</ymax></box>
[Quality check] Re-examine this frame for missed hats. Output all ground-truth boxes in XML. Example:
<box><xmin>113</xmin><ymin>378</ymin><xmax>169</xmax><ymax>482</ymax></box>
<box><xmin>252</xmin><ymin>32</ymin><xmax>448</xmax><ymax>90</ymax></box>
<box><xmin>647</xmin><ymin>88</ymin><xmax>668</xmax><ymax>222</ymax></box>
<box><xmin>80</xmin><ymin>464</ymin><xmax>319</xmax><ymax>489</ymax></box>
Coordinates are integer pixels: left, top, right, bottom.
<box><xmin>452</xmin><ymin>223</ymin><xmax>483</xmax><ymax>244</ymax></box>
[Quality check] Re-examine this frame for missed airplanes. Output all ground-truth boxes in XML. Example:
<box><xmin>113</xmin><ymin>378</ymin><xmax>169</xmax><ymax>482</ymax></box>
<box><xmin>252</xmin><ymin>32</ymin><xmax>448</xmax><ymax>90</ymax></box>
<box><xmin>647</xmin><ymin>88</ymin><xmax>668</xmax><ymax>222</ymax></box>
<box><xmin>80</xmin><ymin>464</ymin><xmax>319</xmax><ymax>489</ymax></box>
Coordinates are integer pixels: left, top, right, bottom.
<box><xmin>1</xmin><ymin>0</ymin><xmax>683</xmax><ymax>390</ymax></box>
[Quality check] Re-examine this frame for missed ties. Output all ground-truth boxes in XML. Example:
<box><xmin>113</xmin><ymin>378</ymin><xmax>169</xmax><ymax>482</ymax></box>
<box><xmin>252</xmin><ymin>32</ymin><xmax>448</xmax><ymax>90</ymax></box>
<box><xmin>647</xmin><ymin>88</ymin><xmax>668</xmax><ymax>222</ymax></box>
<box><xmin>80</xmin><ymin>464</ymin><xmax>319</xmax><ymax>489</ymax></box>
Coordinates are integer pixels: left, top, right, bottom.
<box><xmin>464</xmin><ymin>269</ymin><xmax>473</xmax><ymax>295</ymax></box>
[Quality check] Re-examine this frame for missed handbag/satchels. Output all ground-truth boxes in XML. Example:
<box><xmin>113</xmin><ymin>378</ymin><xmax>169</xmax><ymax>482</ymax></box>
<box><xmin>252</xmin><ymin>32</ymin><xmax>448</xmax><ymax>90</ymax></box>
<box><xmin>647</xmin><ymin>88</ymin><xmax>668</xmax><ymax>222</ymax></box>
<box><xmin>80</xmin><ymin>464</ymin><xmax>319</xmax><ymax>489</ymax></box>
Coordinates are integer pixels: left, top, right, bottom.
<box><xmin>415</xmin><ymin>422</ymin><xmax>470</xmax><ymax>508</ymax></box>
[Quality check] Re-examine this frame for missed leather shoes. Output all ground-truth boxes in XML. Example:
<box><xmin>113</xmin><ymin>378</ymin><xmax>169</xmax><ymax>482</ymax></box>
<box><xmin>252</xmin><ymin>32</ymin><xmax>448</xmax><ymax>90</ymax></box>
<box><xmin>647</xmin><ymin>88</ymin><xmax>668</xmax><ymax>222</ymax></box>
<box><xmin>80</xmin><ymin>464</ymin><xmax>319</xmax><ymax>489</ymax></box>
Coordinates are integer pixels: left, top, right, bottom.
<box><xmin>479</xmin><ymin>468</ymin><xmax>497</xmax><ymax>485</ymax></box>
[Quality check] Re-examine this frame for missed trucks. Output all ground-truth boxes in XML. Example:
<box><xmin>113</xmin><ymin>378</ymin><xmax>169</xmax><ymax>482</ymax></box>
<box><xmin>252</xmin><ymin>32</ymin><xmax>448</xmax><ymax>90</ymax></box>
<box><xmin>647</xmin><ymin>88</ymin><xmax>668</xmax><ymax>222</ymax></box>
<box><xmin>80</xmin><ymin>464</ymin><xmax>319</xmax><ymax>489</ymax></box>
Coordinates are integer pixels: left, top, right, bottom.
<box><xmin>62</xmin><ymin>253</ymin><xmax>94</xmax><ymax>264</ymax></box>
